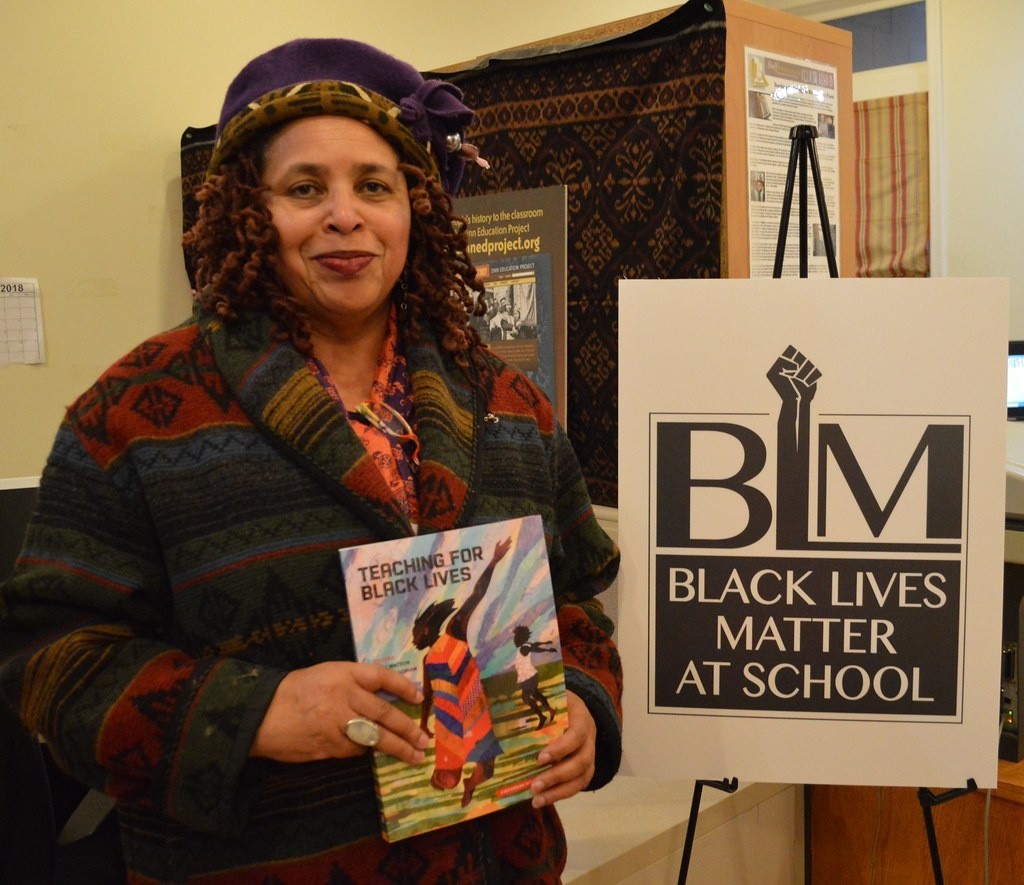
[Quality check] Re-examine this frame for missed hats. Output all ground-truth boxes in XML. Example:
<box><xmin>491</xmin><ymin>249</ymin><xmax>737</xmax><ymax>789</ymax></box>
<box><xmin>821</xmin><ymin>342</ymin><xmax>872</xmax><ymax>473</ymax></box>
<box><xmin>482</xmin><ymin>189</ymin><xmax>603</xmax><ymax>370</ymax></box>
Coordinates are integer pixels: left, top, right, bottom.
<box><xmin>205</xmin><ymin>38</ymin><xmax>491</xmax><ymax>209</ymax></box>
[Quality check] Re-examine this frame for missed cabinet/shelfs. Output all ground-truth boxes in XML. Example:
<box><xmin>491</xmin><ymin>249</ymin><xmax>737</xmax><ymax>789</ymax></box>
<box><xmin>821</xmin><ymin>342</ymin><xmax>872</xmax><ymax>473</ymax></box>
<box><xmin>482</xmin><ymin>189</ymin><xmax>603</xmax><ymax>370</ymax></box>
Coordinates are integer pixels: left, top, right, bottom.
<box><xmin>551</xmin><ymin>771</ymin><xmax>804</xmax><ymax>885</ymax></box>
<box><xmin>804</xmin><ymin>754</ymin><xmax>1024</xmax><ymax>885</ymax></box>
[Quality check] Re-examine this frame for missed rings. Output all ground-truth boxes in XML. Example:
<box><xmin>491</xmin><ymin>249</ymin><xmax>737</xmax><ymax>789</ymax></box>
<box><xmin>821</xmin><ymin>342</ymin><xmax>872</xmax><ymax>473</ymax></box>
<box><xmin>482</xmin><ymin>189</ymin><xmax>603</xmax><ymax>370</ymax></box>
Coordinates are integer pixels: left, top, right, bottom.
<box><xmin>345</xmin><ymin>718</ymin><xmax>381</xmax><ymax>746</ymax></box>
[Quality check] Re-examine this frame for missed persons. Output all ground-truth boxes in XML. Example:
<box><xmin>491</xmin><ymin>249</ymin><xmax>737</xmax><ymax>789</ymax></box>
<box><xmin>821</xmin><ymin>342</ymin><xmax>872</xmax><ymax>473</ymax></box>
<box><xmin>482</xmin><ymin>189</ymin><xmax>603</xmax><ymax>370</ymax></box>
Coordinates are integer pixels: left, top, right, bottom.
<box><xmin>752</xmin><ymin>181</ymin><xmax>765</xmax><ymax>201</ymax></box>
<box><xmin>0</xmin><ymin>40</ymin><xmax>623</xmax><ymax>885</ymax></box>
<box><xmin>818</xmin><ymin>115</ymin><xmax>828</xmax><ymax>137</ymax></box>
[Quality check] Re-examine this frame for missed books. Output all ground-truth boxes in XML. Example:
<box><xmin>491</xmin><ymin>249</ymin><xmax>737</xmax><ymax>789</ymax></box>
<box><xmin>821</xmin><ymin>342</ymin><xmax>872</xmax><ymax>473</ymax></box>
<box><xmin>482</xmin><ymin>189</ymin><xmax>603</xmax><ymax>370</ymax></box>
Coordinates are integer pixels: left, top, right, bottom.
<box><xmin>339</xmin><ymin>514</ymin><xmax>569</xmax><ymax>843</ymax></box>
